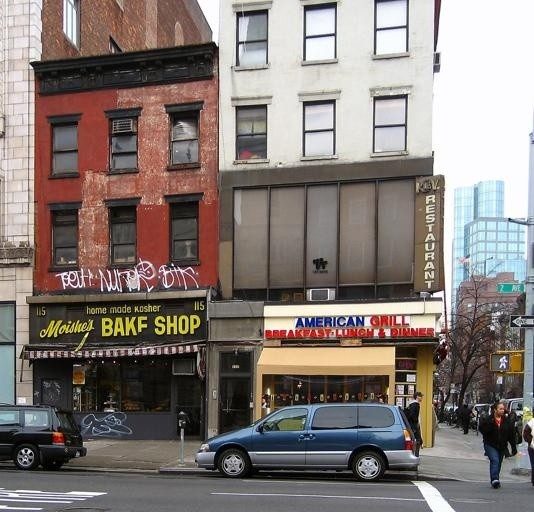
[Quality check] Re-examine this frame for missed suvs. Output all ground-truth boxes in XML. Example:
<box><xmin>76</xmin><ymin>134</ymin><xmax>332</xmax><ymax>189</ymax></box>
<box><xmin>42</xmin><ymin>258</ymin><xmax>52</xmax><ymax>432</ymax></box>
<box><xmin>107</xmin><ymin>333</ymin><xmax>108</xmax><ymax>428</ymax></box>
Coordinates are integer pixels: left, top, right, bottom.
<box><xmin>0</xmin><ymin>402</ymin><xmax>87</xmax><ymax>472</ymax></box>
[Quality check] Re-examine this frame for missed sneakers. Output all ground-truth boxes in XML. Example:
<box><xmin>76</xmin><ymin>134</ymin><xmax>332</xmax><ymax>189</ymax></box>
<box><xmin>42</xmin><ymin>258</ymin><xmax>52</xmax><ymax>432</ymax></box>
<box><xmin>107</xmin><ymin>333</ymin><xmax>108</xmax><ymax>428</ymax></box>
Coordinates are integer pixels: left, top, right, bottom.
<box><xmin>492</xmin><ymin>480</ymin><xmax>501</xmax><ymax>488</ymax></box>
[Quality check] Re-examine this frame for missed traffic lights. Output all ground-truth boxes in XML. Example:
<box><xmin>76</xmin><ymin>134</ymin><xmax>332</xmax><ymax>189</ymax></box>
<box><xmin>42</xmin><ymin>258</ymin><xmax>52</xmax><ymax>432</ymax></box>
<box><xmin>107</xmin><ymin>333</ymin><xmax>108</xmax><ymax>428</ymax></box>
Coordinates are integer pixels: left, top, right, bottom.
<box><xmin>489</xmin><ymin>353</ymin><xmax>511</xmax><ymax>373</ymax></box>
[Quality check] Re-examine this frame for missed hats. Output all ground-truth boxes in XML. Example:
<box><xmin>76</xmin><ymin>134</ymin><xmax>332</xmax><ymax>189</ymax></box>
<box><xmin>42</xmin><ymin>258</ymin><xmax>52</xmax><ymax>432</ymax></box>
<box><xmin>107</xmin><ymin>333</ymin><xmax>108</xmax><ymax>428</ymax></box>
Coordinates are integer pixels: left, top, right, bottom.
<box><xmin>414</xmin><ymin>392</ymin><xmax>424</xmax><ymax>397</ymax></box>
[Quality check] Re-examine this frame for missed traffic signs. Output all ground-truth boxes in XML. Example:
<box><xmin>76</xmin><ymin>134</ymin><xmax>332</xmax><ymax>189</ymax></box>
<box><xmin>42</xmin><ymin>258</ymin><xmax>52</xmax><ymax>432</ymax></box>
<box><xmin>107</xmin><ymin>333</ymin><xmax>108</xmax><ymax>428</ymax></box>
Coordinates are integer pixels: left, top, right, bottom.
<box><xmin>495</xmin><ymin>282</ymin><xmax>526</xmax><ymax>292</ymax></box>
<box><xmin>509</xmin><ymin>314</ymin><xmax>534</xmax><ymax>328</ymax></box>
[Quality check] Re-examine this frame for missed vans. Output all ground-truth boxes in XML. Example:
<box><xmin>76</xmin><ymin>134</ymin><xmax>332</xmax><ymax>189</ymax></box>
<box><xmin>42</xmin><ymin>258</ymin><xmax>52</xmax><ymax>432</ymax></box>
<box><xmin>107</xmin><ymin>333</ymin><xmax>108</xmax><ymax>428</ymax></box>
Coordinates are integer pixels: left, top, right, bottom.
<box><xmin>194</xmin><ymin>401</ymin><xmax>421</xmax><ymax>484</ymax></box>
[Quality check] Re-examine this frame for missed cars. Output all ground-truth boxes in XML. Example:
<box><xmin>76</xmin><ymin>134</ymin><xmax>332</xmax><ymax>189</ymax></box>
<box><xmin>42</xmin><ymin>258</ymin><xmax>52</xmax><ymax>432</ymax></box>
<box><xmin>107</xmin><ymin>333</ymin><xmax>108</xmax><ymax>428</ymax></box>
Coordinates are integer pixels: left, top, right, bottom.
<box><xmin>446</xmin><ymin>396</ymin><xmax>534</xmax><ymax>429</ymax></box>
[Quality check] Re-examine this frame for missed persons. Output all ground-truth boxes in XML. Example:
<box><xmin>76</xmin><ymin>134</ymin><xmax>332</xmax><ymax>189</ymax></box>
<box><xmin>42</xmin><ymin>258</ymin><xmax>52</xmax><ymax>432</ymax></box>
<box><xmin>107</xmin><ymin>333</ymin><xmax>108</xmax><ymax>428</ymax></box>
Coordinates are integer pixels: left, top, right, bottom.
<box><xmin>434</xmin><ymin>397</ymin><xmax>445</xmax><ymax>412</ymax></box>
<box><xmin>522</xmin><ymin>402</ymin><xmax>534</xmax><ymax>486</ymax></box>
<box><xmin>403</xmin><ymin>391</ymin><xmax>426</xmax><ymax>458</ymax></box>
<box><xmin>499</xmin><ymin>398</ymin><xmax>511</xmax><ymax>459</ymax></box>
<box><xmin>262</xmin><ymin>393</ymin><xmax>269</xmax><ymax>409</ymax></box>
<box><xmin>460</xmin><ymin>402</ymin><xmax>474</xmax><ymax>435</ymax></box>
<box><xmin>508</xmin><ymin>410</ymin><xmax>522</xmax><ymax>455</ymax></box>
<box><xmin>477</xmin><ymin>402</ymin><xmax>514</xmax><ymax>489</ymax></box>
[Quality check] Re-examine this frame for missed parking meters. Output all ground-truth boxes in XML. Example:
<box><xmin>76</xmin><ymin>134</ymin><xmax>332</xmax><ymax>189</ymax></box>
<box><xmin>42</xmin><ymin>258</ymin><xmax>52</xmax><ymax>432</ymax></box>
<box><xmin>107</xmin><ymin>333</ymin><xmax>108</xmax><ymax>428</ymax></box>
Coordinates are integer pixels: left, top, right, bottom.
<box><xmin>177</xmin><ymin>410</ymin><xmax>189</xmax><ymax>468</ymax></box>
